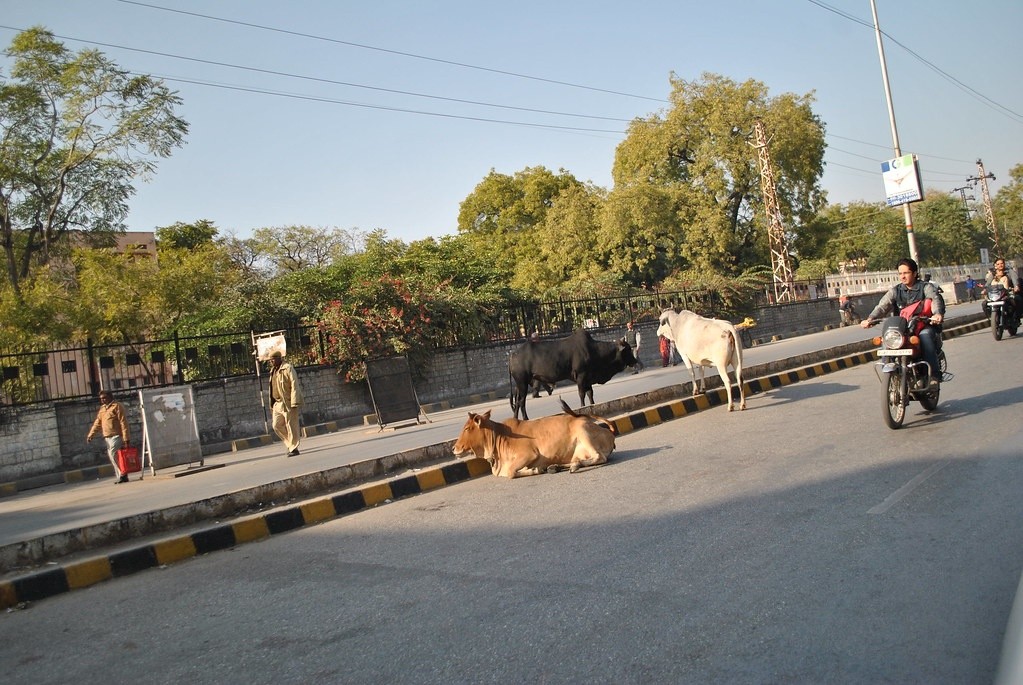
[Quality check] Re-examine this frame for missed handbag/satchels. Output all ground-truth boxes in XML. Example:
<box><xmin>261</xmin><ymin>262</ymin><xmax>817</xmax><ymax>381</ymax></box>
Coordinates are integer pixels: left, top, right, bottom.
<box><xmin>117</xmin><ymin>446</ymin><xmax>141</xmax><ymax>475</ymax></box>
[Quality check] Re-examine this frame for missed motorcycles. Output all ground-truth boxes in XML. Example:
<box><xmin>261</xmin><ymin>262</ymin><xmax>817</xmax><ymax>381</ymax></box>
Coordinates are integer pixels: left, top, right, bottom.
<box><xmin>867</xmin><ymin>317</ymin><xmax>956</xmax><ymax>429</ymax></box>
<box><xmin>979</xmin><ymin>285</ymin><xmax>1023</xmax><ymax>341</ymax></box>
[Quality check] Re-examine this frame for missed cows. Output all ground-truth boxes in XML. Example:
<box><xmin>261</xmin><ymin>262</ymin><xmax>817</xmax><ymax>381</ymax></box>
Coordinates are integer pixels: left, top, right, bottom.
<box><xmin>451</xmin><ymin>330</ymin><xmax>638</xmax><ymax>477</ymax></box>
<box><xmin>656</xmin><ymin>309</ymin><xmax>746</xmax><ymax>412</ymax></box>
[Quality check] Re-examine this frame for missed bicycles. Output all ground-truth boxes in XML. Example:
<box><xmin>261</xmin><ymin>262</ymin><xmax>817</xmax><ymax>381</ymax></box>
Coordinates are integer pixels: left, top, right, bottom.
<box><xmin>844</xmin><ymin>304</ymin><xmax>861</xmax><ymax>326</ymax></box>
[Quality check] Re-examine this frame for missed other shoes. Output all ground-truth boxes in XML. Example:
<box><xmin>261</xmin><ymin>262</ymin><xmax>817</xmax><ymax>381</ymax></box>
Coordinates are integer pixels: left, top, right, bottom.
<box><xmin>631</xmin><ymin>372</ymin><xmax>638</xmax><ymax>374</ymax></box>
<box><xmin>533</xmin><ymin>395</ymin><xmax>542</xmax><ymax>398</ymax></box>
<box><xmin>930</xmin><ymin>378</ymin><xmax>939</xmax><ymax>391</ymax></box>
<box><xmin>549</xmin><ymin>388</ymin><xmax>553</xmax><ymax>396</ymax></box>
<box><xmin>287</xmin><ymin>449</ymin><xmax>299</xmax><ymax>458</ymax></box>
<box><xmin>114</xmin><ymin>476</ymin><xmax>129</xmax><ymax>484</ymax></box>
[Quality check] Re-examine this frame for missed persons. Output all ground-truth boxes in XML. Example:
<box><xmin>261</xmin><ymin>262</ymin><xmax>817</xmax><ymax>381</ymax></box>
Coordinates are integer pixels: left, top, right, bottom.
<box><xmin>840</xmin><ymin>295</ymin><xmax>860</xmax><ymax>322</ymax></box>
<box><xmin>659</xmin><ymin>335</ymin><xmax>680</xmax><ymax>368</ymax></box>
<box><xmin>268</xmin><ymin>352</ymin><xmax>302</xmax><ymax>456</ymax></box>
<box><xmin>964</xmin><ymin>275</ymin><xmax>978</xmax><ymax>304</ymax></box>
<box><xmin>87</xmin><ymin>390</ymin><xmax>129</xmax><ymax>485</ymax></box>
<box><xmin>531</xmin><ymin>332</ymin><xmax>553</xmax><ymax>398</ymax></box>
<box><xmin>860</xmin><ymin>258</ymin><xmax>945</xmax><ymax>384</ymax></box>
<box><xmin>977</xmin><ymin>258</ymin><xmax>1019</xmax><ymax>329</ymax></box>
<box><xmin>625</xmin><ymin>322</ymin><xmax>645</xmax><ymax>374</ymax></box>
